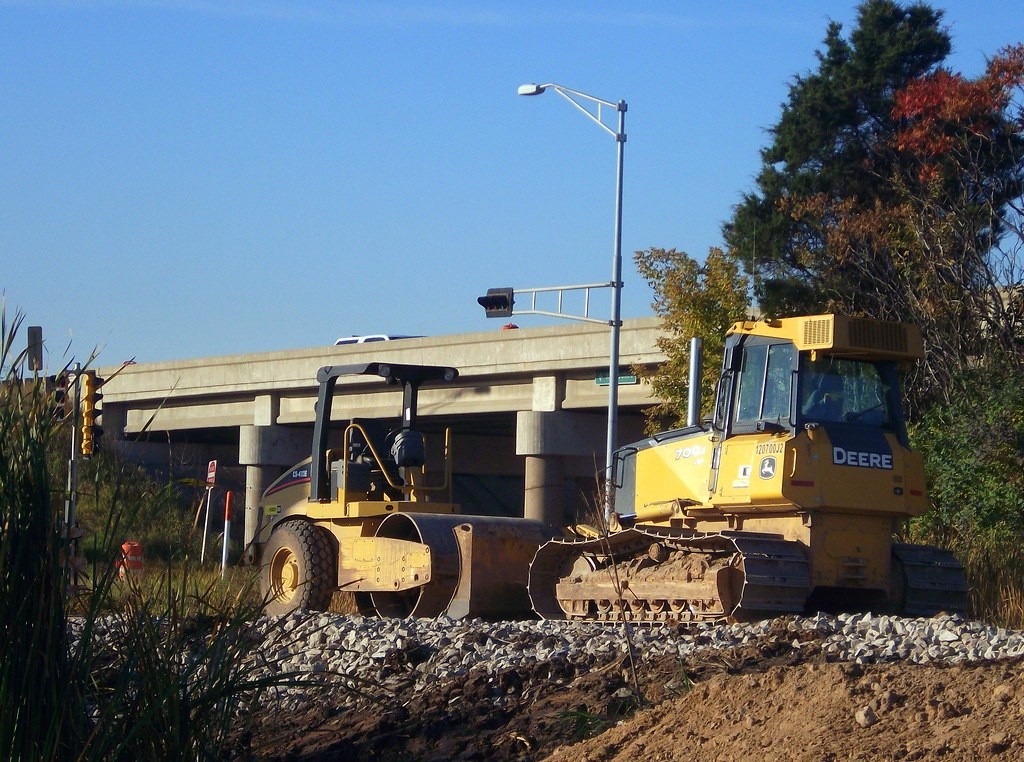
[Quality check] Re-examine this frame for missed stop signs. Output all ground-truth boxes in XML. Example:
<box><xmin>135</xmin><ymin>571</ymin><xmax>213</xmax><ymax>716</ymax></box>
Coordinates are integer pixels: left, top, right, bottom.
<box><xmin>205</xmin><ymin>459</ymin><xmax>218</xmax><ymax>490</ymax></box>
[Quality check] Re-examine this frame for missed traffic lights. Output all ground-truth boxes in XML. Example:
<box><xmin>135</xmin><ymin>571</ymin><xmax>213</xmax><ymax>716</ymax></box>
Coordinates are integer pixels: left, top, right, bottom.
<box><xmin>81</xmin><ymin>370</ymin><xmax>104</xmax><ymax>459</ymax></box>
<box><xmin>53</xmin><ymin>374</ymin><xmax>69</xmax><ymax>421</ymax></box>
<box><xmin>477</xmin><ymin>287</ymin><xmax>514</xmax><ymax>318</ymax></box>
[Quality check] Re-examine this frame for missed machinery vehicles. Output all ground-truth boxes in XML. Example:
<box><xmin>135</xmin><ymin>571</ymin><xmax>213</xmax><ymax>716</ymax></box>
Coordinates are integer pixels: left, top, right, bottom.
<box><xmin>242</xmin><ymin>360</ymin><xmax>574</xmax><ymax>623</ymax></box>
<box><xmin>525</xmin><ymin>312</ymin><xmax>970</xmax><ymax>629</ymax></box>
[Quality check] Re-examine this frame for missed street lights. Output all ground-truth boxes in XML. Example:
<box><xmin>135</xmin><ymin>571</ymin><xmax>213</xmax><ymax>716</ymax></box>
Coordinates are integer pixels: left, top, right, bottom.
<box><xmin>516</xmin><ymin>82</ymin><xmax>630</xmax><ymax>540</ymax></box>
